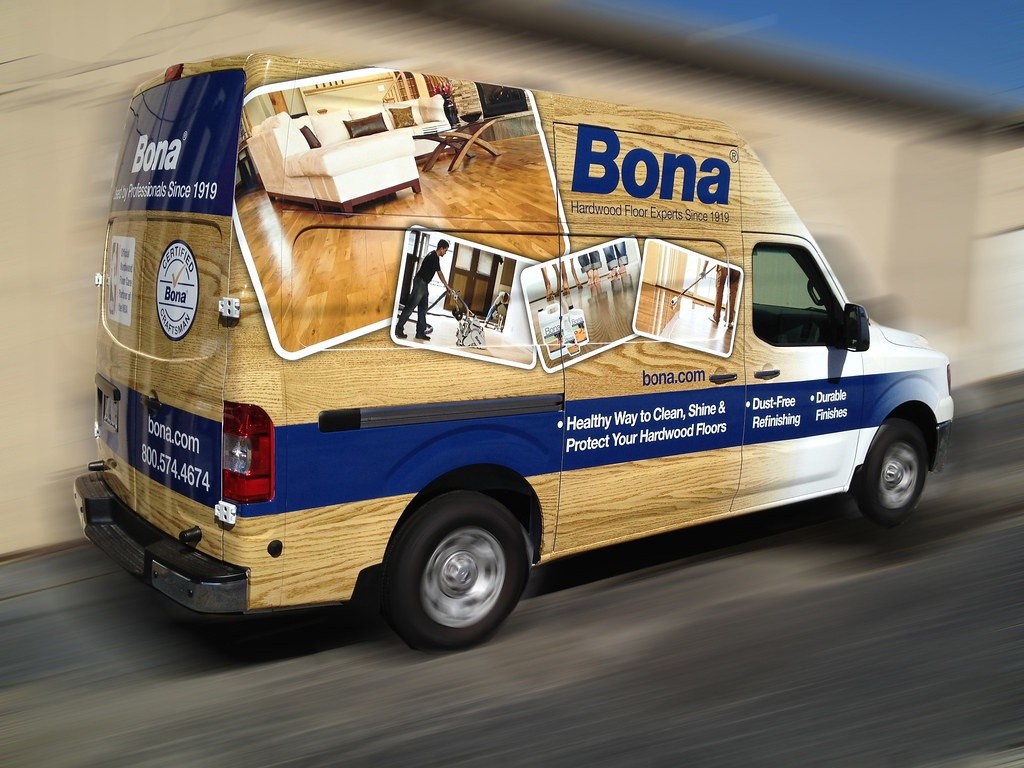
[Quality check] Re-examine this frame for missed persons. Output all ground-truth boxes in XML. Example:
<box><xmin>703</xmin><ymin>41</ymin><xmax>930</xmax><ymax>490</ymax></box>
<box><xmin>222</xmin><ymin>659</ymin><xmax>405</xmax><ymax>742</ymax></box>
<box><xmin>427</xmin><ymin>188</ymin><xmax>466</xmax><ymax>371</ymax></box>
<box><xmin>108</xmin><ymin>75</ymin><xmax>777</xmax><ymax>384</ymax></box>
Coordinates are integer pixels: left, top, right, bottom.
<box><xmin>541</xmin><ymin>241</ymin><xmax>629</xmax><ymax>300</ymax></box>
<box><xmin>698</xmin><ymin>260</ymin><xmax>740</xmax><ymax>328</ymax></box>
<box><xmin>395</xmin><ymin>239</ymin><xmax>453</xmax><ymax>340</ymax></box>
<box><xmin>483</xmin><ymin>292</ymin><xmax>510</xmax><ymax>328</ymax></box>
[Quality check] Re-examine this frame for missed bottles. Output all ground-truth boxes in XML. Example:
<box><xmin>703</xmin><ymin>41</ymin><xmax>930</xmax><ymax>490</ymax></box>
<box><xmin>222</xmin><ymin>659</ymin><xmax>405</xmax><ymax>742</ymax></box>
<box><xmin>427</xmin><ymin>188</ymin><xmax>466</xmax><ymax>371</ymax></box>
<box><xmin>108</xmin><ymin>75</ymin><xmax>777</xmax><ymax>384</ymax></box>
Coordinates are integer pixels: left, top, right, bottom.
<box><xmin>568</xmin><ymin>305</ymin><xmax>589</xmax><ymax>346</ymax></box>
<box><xmin>538</xmin><ymin>303</ymin><xmax>568</xmax><ymax>360</ymax></box>
<box><xmin>563</xmin><ymin>315</ymin><xmax>580</xmax><ymax>356</ymax></box>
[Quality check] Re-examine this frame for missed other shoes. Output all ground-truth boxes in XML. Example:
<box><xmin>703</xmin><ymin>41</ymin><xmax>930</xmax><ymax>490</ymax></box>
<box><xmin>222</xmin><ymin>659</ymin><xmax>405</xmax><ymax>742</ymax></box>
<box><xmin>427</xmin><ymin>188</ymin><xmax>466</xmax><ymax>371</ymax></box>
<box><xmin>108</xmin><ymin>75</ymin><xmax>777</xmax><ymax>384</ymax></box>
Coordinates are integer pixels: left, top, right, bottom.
<box><xmin>709</xmin><ymin>317</ymin><xmax>718</xmax><ymax>324</ymax></box>
<box><xmin>724</xmin><ymin>322</ymin><xmax>733</xmax><ymax>327</ymax></box>
<box><xmin>416</xmin><ymin>334</ymin><xmax>431</xmax><ymax>340</ymax></box>
<box><xmin>395</xmin><ymin>330</ymin><xmax>407</xmax><ymax>337</ymax></box>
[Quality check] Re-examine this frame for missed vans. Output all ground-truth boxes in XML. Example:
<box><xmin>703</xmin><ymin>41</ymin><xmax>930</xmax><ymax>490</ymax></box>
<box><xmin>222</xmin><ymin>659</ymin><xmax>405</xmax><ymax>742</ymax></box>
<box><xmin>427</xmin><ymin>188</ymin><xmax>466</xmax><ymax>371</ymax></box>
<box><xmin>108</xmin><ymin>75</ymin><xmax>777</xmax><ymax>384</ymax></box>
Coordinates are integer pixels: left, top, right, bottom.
<box><xmin>74</xmin><ymin>47</ymin><xmax>958</xmax><ymax>647</ymax></box>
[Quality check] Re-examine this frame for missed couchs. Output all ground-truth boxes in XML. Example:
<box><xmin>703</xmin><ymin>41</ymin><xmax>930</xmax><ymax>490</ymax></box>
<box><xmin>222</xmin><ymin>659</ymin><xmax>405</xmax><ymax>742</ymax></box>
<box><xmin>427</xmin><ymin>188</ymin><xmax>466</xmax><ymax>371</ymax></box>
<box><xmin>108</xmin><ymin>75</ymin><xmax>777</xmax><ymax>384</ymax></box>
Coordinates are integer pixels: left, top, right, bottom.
<box><xmin>246</xmin><ymin>96</ymin><xmax>452</xmax><ymax>218</ymax></box>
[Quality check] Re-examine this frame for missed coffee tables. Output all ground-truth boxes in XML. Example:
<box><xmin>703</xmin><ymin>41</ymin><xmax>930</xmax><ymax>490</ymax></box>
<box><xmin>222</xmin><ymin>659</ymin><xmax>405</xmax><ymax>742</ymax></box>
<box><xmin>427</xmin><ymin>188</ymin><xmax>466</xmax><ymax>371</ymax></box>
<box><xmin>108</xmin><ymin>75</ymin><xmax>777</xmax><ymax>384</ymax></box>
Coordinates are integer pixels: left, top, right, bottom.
<box><xmin>413</xmin><ymin>116</ymin><xmax>504</xmax><ymax>172</ymax></box>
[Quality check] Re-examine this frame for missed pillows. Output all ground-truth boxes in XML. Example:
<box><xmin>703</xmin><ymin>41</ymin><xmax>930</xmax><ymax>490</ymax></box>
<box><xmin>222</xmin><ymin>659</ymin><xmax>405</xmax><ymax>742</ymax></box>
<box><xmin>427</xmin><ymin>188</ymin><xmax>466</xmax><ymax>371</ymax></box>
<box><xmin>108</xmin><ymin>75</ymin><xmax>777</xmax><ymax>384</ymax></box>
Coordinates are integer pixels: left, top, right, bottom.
<box><xmin>343</xmin><ymin>112</ymin><xmax>389</xmax><ymax>139</ymax></box>
<box><xmin>299</xmin><ymin>125</ymin><xmax>321</xmax><ymax>148</ymax></box>
<box><xmin>389</xmin><ymin>107</ymin><xmax>418</xmax><ymax>129</ymax></box>
